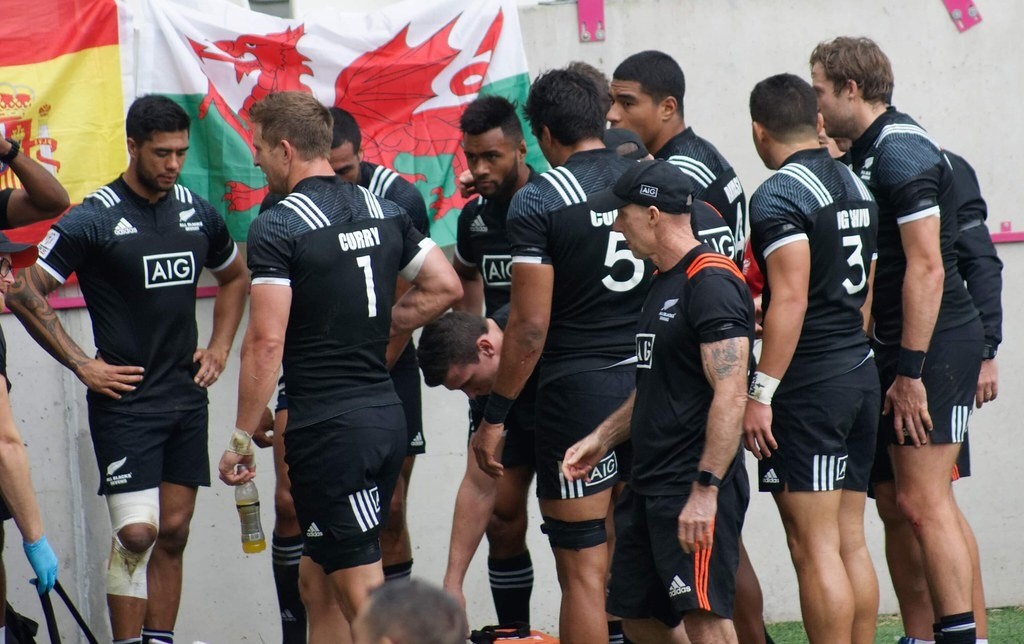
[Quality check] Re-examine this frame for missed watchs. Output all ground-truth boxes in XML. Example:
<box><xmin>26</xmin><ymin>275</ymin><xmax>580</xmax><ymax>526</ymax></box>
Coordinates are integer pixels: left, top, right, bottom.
<box><xmin>693</xmin><ymin>470</ymin><xmax>722</xmax><ymax>487</ymax></box>
<box><xmin>0</xmin><ymin>138</ymin><xmax>20</xmax><ymax>162</ymax></box>
<box><xmin>982</xmin><ymin>344</ymin><xmax>998</xmax><ymax>361</ymax></box>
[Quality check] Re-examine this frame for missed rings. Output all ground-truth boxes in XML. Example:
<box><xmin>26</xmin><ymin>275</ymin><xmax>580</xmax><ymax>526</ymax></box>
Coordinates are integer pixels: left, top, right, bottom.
<box><xmin>985</xmin><ymin>392</ymin><xmax>992</xmax><ymax>396</ymax></box>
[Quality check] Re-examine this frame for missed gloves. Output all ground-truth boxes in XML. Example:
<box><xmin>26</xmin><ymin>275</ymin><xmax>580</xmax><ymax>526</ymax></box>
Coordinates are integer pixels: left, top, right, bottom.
<box><xmin>23</xmin><ymin>539</ymin><xmax>58</xmax><ymax>597</ymax></box>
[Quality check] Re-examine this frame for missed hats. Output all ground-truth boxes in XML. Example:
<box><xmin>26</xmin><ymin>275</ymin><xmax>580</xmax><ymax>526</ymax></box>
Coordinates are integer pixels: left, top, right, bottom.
<box><xmin>586</xmin><ymin>160</ymin><xmax>694</xmax><ymax>215</ymax></box>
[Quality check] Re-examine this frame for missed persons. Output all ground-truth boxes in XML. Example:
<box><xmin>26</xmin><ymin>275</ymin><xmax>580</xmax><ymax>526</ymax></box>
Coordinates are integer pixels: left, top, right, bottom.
<box><xmin>0</xmin><ymin>37</ymin><xmax>1003</xmax><ymax>644</ymax></box>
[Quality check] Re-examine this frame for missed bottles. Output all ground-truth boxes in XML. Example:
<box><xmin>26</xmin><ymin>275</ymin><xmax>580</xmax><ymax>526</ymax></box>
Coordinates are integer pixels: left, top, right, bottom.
<box><xmin>235</xmin><ymin>466</ymin><xmax>266</xmax><ymax>553</ymax></box>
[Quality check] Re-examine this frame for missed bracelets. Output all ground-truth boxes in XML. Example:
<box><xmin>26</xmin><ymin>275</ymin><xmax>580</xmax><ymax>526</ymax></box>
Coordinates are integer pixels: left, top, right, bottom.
<box><xmin>897</xmin><ymin>347</ymin><xmax>928</xmax><ymax>379</ymax></box>
<box><xmin>747</xmin><ymin>371</ymin><xmax>781</xmax><ymax>405</ymax></box>
<box><xmin>483</xmin><ymin>390</ymin><xmax>514</xmax><ymax>424</ymax></box>
